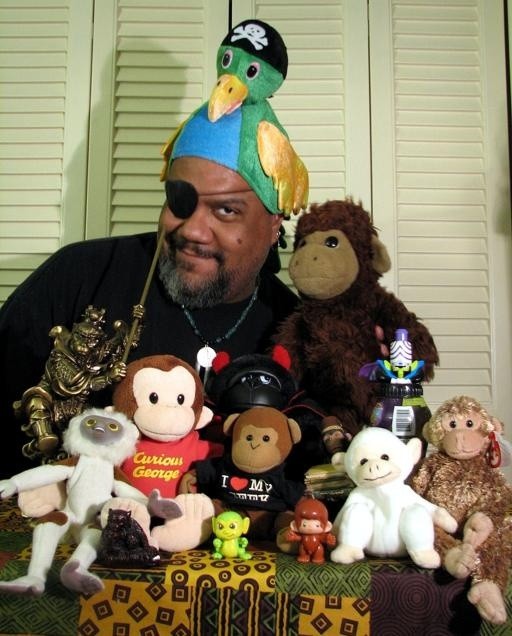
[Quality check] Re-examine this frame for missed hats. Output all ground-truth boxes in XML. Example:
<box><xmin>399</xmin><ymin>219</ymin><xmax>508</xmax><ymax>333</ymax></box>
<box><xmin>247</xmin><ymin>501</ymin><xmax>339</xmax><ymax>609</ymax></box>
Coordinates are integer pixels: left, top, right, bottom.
<box><xmin>158</xmin><ymin>19</ymin><xmax>310</xmax><ymax>219</ymax></box>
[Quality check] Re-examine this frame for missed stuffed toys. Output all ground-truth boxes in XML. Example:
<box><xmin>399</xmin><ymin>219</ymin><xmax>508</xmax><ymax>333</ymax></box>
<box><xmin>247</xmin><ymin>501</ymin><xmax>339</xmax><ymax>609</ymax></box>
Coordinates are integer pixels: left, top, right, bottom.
<box><xmin>0</xmin><ymin>344</ymin><xmax>512</xmax><ymax>624</ymax></box>
<box><xmin>270</xmin><ymin>199</ymin><xmax>438</xmax><ymax>426</ymax></box>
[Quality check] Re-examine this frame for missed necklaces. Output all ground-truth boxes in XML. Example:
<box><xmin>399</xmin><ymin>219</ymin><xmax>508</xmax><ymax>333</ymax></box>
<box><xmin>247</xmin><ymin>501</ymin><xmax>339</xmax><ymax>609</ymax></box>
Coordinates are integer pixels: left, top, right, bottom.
<box><xmin>181</xmin><ymin>285</ymin><xmax>263</xmax><ymax>368</ymax></box>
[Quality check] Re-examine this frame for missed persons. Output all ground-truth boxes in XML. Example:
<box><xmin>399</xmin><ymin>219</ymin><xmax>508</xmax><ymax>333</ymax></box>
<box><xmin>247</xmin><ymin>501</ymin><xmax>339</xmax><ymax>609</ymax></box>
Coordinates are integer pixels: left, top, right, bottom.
<box><xmin>1</xmin><ymin>103</ymin><xmax>292</xmax><ymax>476</ymax></box>
